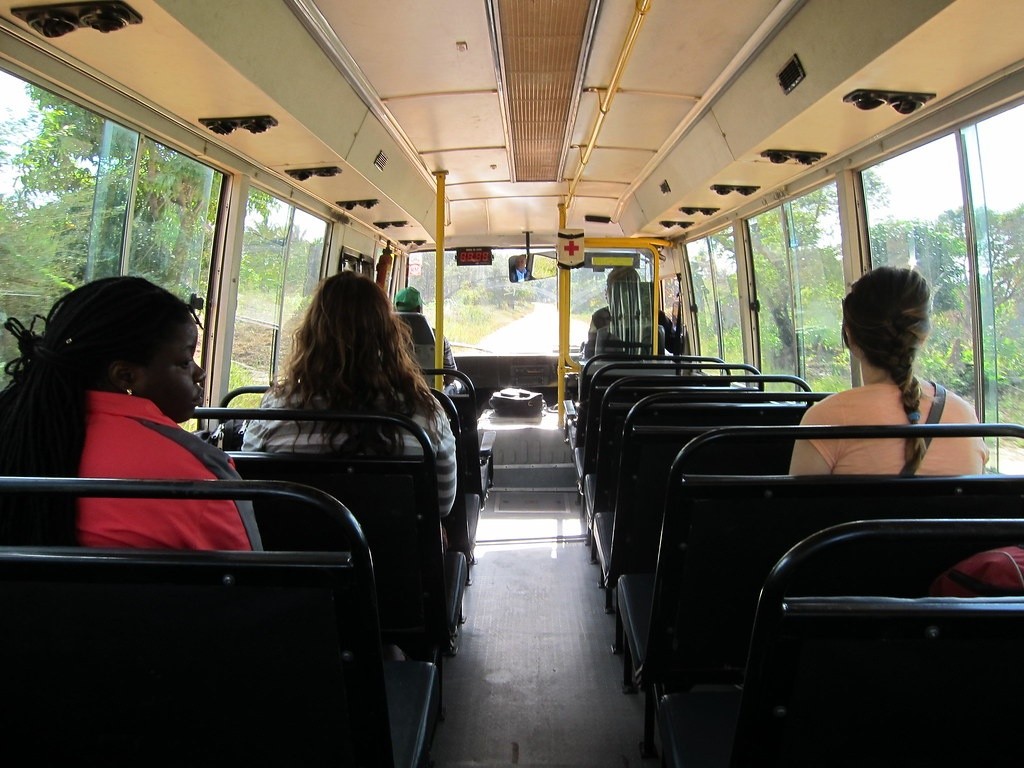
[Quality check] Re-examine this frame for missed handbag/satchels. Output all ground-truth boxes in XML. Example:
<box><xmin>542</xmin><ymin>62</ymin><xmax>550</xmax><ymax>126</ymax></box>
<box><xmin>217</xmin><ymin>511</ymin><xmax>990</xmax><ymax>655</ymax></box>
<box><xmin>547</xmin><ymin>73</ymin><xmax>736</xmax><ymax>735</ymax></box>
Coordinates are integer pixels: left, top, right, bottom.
<box><xmin>937</xmin><ymin>544</ymin><xmax>1024</xmax><ymax>597</ymax></box>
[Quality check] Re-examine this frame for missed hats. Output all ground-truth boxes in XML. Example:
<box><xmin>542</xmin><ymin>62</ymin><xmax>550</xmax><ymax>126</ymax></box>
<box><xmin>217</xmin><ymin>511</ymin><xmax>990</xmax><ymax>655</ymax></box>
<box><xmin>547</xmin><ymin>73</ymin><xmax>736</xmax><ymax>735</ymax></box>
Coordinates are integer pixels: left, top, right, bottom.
<box><xmin>396</xmin><ymin>287</ymin><xmax>419</xmax><ymax>311</ymax></box>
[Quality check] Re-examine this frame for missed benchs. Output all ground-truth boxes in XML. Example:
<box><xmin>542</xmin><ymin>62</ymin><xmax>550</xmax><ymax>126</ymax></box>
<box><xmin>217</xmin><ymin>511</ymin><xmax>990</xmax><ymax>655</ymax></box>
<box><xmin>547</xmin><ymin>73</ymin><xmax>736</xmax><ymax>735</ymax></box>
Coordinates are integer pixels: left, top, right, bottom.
<box><xmin>563</xmin><ymin>355</ymin><xmax>1024</xmax><ymax>768</ymax></box>
<box><xmin>0</xmin><ymin>370</ymin><xmax>495</xmax><ymax>768</ymax></box>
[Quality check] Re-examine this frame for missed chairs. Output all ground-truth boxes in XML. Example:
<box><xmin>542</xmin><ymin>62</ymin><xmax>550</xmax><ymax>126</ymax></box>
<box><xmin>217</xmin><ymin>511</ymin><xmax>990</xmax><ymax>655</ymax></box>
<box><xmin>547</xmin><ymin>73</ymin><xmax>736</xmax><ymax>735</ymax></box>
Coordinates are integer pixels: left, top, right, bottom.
<box><xmin>595</xmin><ymin>281</ymin><xmax>665</xmax><ymax>356</ymax></box>
<box><xmin>391</xmin><ymin>312</ymin><xmax>436</xmax><ymax>388</ymax></box>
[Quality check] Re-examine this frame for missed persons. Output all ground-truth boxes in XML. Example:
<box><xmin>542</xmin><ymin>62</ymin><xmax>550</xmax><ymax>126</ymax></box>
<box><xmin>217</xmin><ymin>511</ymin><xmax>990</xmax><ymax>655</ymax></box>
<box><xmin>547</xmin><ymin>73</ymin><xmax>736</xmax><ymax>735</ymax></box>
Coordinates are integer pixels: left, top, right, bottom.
<box><xmin>789</xmin><ymin>265</ymin><xmax>990</xmax><ymax>477</ymax></box>
<box><xmin>0</xmin><ymin>275</ymin><xmax>265</xmax><ymax>552</ymax></box>
<box><xmin>510</xmin><ymin>254</ymin><xmax>536</xmax><ymax>283</ymax></box>
<box><xmin>241</xmin><ymin>270</ymin><xmax>457</xmax><ymax>662</ymax></box>
<box><xmin>584</xmin><ymin>267</ymin><xmax>685</xmax><ymax>361</ymax></box>
<box><xmin>394</xmin><ymin>287</ymin><xmax>467</xmax><ymax>395</ymax></box>
